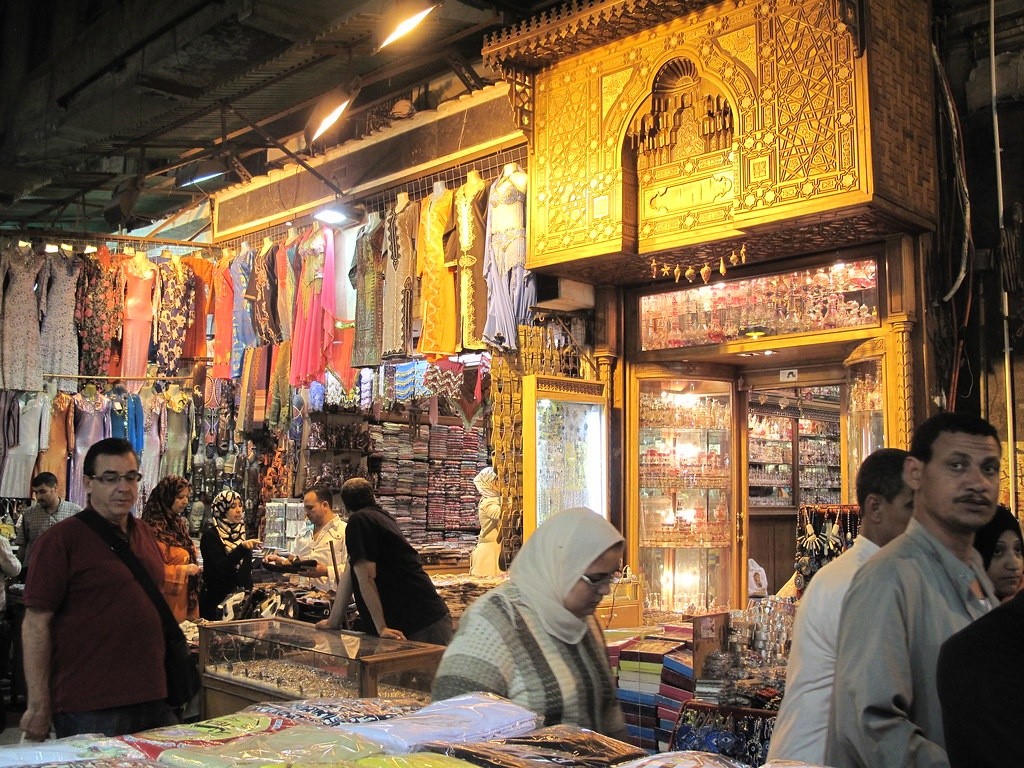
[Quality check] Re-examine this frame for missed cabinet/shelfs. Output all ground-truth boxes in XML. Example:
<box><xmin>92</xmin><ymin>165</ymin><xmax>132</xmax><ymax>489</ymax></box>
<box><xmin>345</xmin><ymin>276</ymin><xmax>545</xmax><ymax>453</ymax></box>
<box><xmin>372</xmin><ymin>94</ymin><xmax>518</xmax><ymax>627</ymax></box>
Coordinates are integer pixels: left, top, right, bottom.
<box><xmin>296</xmin><ymin>411</ymin><xmax>491</xmax><ymax>567</ymax></box>
<box><xmin>748</xmin><ymin>402</ymin><xmax>840</xmax><ymax>508</ymax></box>
<box><xmin>637</xmin><ymin>383</ymin><xmax>735</xmax><ymax>626</ymax></box>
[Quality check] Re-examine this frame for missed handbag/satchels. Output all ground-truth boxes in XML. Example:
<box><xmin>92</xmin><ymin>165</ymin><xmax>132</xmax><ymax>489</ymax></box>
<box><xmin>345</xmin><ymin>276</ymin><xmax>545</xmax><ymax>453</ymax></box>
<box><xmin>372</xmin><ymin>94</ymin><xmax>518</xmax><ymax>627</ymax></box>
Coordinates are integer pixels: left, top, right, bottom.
<box><xmin>163</xmin><ymin>627</ymin><xmax>201</xmax><ymax>706</ymax></box>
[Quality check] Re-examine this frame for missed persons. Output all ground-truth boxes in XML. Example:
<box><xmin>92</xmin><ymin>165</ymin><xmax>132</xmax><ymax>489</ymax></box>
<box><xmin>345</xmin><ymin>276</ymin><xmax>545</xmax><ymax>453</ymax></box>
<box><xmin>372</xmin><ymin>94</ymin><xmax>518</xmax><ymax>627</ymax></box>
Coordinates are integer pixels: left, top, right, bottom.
<box><xmin>14</xmin><ymin>472</ymin><xmax>84</xmax><ymax>568</ymax></box>
<box><xmin>266</xmin><ymin>486</ymin><xmax>348</xmax><ymax>593</ymax></box>
<box><xmin>0</xmin><ymin>535</ymin><xmax>22</xmax><ymax>616</ymax></box>
<box><xmin>766</xmin><ymin>448</ymin><xmax>914</xmax><ymax>768</ymax></box>
<box><xmin>937</xmin><ymin>582</ymin><xmax>1024</xmax><ymax>768</ymax></box>
<box><xmin>0</xmin><ymin>163</ymin><xmax>539</xmax><ymax>518</ymax></box>
<box><xmin>825</xmin><ymin>413</ymin><xmax>1000</xmax><ymax>768</ymax></box>
<box><xmin>141</xmin><ymin>475</ymin><xmax>200</xmax><ymax>624</ymax></box>
<box><xmin>198</xmin><ymin>490</ymin><xmax>264</xmax><ymax>621</ymax></box>
<box><xmin>21</xmin><ymin>439</ymin><xmax>180</xmax><ymax>742</ymax></box>
<box><xmin>470</xmin><ymin>467</ymin><xmax>499</xmax><ymax>575</ymax></box>
<box><xmin>974</xmin><ymin>504</ymin><xmax>1024</xmax><ymax>604</ymax></box>
<box><xmin>315</xmin><ymin>478</ymin><xmax>452</xmax><ymax>692</ymax></box>
<box><xmin>430</xmin><ymin>507</ymin><xmax>626</xmax><ymax>742</ymax></box>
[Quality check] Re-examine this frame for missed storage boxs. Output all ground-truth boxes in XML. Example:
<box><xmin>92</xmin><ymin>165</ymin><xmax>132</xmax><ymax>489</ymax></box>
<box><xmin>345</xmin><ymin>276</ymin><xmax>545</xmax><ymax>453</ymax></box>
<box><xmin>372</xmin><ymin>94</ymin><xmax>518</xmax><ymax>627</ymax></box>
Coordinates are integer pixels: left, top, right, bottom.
<box><xmin>603</xmin><ymin>624</ymin><xmax>694</xmax><ymax>753</ymax></box>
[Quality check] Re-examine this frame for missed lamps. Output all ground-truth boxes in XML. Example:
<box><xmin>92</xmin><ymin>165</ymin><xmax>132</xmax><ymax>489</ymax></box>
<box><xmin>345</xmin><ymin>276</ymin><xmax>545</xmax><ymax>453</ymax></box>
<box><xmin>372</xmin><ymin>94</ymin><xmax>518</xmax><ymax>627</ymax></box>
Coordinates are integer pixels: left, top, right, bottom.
<box><xmin>173</xmin><ymin>160</ymin><xmax>232</xmax><ymax>188</ymax></box>
<box><xmin>104</xmin><ymin>176</ymin><xmax>144</xmax><ymax>226</ymax></box>
<box><xmin>372</xmin><ymin>1</ymin><xmax>440</xmax><ymax>55</ymax></box>
<box><xmin>312</xmin><ymin>205</ymin><xmax>363</xmax><ymax>229</ymax></box>
<box><xmin>301</xmin><ymin>85</ymin><xmax>365</xmax><ymax>152</ymax></box>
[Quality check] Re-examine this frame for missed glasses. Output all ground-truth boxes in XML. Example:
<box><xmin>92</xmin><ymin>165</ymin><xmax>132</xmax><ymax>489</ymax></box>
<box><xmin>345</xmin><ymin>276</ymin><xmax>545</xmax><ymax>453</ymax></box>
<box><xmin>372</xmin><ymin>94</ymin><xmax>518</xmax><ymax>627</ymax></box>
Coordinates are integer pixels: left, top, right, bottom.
<box><xmin>87</xmin><ymin>472</ymin><xmax>143</xmax><ymax>485</ymax></box>
<box><xmin>581</xmin><ymin>575</ymin><xmax>618</xmax><ymax>592</ymax></box>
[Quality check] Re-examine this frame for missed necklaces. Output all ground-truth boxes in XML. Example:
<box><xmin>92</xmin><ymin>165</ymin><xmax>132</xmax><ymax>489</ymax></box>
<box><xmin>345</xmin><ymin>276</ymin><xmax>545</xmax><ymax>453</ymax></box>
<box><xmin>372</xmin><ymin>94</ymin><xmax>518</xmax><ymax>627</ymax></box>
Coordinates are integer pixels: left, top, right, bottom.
<box><xmin>794</xmin><ymin>504</ymin><xmax>862</xmax><ymax>591</ymax></box>
